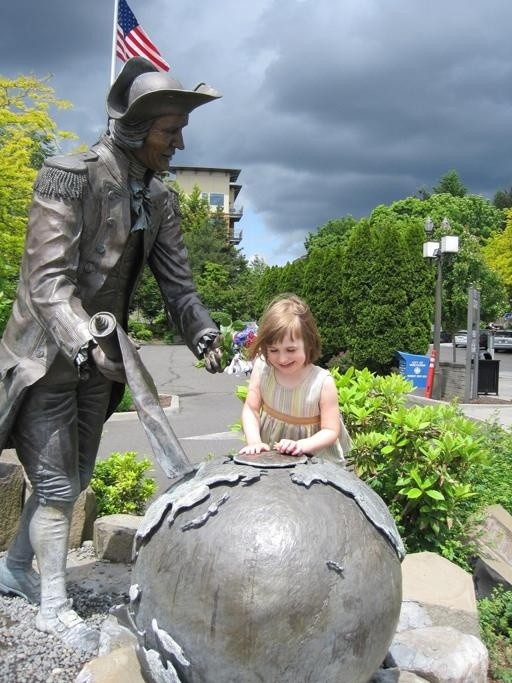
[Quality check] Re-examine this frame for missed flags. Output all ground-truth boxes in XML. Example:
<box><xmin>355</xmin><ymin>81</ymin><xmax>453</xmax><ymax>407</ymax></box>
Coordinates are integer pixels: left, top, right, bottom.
<box><xmin>115</xmin><ymin>0</ymin><xmax>170</xmax><ymax>76</ymax></box>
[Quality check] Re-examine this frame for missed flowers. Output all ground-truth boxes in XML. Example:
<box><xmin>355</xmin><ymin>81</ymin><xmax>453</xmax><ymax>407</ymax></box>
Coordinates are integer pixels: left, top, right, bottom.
<box><xmin>193</xmin><ymin>322</ymin><xmax>259</xmax><ymax>374</ymax></box>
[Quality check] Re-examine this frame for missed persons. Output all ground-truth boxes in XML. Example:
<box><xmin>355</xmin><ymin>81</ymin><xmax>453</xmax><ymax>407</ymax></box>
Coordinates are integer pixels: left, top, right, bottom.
<box><xmin>239</xmin><ymin>291</ymin><xmax>352</xmax><ymax>471</ymax></box>
<box><xmin>0</xmin><ymin>54</ymin><xmax>238</xmax><ymax>653</ymax></box>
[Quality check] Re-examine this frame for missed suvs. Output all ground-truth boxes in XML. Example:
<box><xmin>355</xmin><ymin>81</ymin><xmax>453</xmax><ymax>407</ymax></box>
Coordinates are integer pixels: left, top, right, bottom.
<box><xmin>479</xmin><ymin>329</ymin><xmax>492</xmax><ymax>348</ymax></box>
<box><xmin>455</xmin><ymin>329</ymin><xmax>475</xmax><ymax>347</ymax></box>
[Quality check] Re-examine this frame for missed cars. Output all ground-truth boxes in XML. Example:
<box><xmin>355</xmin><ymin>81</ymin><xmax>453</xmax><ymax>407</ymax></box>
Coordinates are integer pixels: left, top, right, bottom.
<box><xmin>493</xmin><ymin>329</ymin><xmax>512</xmax><ymax>351</ymax></box>
<box><xmin>503</xmin><ymin>312</ymin><xmax>512</xmax><ymax>319</ymax></box>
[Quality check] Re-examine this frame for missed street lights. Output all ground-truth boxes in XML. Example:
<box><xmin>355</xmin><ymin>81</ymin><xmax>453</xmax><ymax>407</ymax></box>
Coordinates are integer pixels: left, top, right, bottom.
<box><xmin>422</xmin><ymin>215</ymin><xmax>459</xmax><ymax>400</ymax></box>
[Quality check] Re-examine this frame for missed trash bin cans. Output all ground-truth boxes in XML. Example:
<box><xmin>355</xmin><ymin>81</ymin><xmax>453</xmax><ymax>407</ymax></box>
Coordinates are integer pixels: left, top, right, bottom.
<box><xmin>471</xmin><ymin>360</ymin><xmax>500</xmax><ymax>396</ymax></box>
